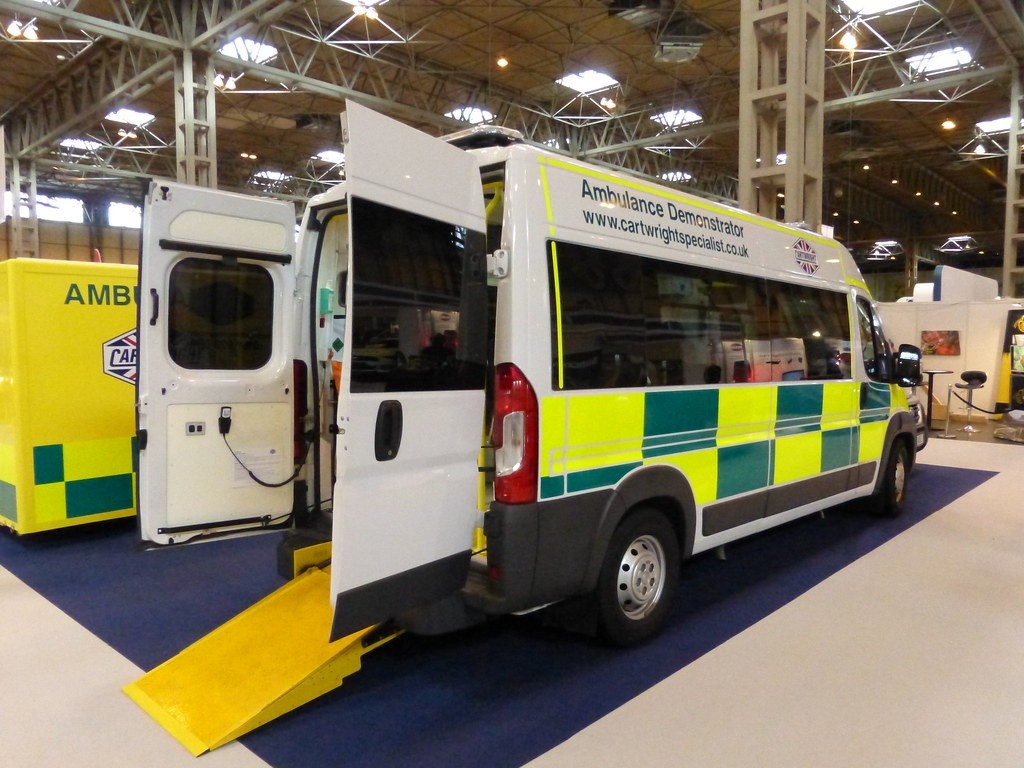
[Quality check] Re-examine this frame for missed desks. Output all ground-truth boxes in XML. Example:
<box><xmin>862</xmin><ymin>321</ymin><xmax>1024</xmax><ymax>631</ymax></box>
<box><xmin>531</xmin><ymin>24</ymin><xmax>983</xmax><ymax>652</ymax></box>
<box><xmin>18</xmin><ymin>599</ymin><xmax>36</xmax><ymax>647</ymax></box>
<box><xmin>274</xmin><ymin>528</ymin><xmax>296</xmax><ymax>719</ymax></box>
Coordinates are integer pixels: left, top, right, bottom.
<box><xmin>923</xmin><ymin>370</ymin><xmax>954</xmax><ymax>431</ymax></box>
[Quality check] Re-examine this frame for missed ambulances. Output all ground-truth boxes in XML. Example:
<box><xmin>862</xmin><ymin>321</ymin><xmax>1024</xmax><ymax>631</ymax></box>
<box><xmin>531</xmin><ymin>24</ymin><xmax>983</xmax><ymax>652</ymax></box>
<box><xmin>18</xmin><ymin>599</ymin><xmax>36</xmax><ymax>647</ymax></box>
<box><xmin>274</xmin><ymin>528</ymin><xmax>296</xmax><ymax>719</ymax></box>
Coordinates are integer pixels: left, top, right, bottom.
<box><xmin>130</xmin><ymin>98</ymin><xmax>921</xmax><ymax>650</ymax></box>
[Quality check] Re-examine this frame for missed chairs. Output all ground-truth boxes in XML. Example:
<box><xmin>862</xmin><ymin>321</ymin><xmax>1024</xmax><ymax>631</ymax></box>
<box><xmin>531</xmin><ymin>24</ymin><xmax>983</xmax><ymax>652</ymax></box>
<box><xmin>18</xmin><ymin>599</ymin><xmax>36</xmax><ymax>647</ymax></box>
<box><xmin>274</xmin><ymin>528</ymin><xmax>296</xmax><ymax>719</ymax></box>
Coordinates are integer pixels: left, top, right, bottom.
<box><xmin>561</xmin><ymin>313</ymin><xmax>751</xmax><ymax>391</ymax></box>
<box><xmin>955</xmin><ymin>371</ymin><xmax>987</xmax><ymax>432</ymax></box>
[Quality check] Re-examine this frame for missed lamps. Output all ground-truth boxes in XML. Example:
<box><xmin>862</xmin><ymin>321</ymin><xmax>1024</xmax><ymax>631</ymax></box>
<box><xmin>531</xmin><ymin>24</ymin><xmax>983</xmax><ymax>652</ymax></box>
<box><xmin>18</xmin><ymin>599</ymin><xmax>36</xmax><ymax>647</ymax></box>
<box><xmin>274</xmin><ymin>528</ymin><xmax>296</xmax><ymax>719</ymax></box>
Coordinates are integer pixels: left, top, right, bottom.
<box><xmin>608</xmin><ymin>0</ymin><xmax>667</xmax><ymax>27</ymax></box>
<box><xmin>296</xmin><ymin>114</ymin><xmax>337</xmax><ymax>130</ymax></box>
<box><xmin>653</xmin><ymin>33</ymin><xmax>704</xmax><ymax>62</ymax></box>
